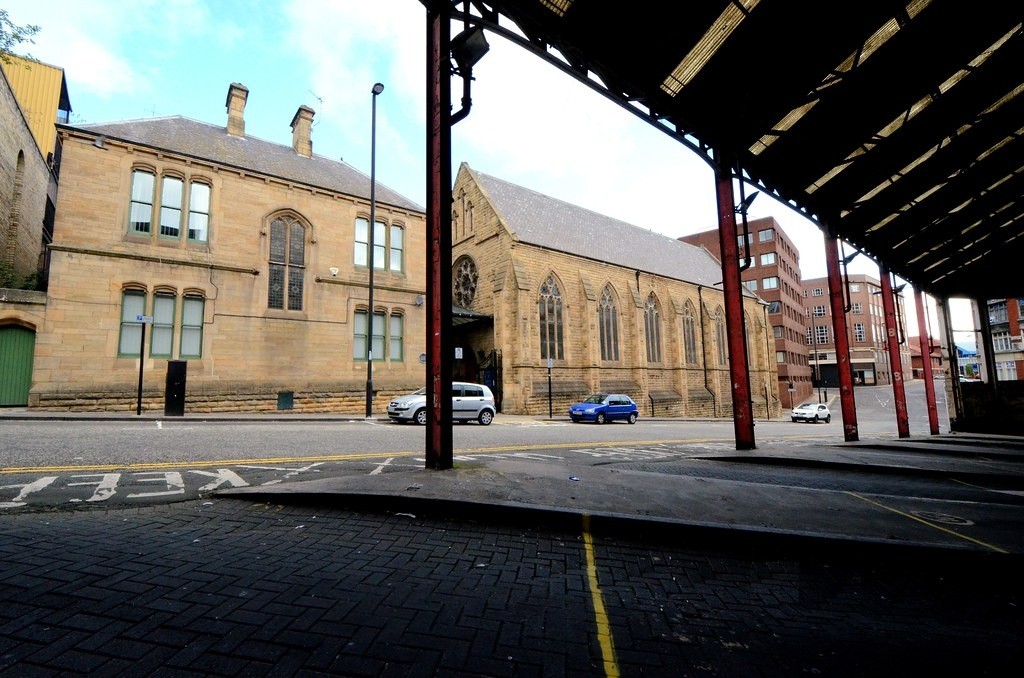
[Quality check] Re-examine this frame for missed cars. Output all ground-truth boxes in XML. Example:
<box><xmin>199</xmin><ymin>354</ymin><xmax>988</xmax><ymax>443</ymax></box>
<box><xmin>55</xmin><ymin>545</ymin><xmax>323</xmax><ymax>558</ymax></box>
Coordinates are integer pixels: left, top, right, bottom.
<box><xmin>386</xmin><ymin>382</ymin><xmax>497</xmax><ymax>426</ymax></box>
<box><xmin>791</xmin><ymin>403</ymin><xmax>831</xmax><ymax>423</ymax></box>
<box><xmin>569</xmin><ymin>394</ymin><xmax>639</xmax><ymax>425</ymax></box>
<box><xmin>934</xmin><ymin>374</ymin><xmax>944</xmax><ymax>380</ymax></box>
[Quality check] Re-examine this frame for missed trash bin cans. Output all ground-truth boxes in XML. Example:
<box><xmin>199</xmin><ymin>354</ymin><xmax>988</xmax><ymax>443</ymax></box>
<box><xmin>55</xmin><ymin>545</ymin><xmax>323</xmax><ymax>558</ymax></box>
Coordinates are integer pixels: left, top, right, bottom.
<box><xmin>164</xmin><ymin>359</ymin><xmax>186</xmax><ymax>416</ymax></box>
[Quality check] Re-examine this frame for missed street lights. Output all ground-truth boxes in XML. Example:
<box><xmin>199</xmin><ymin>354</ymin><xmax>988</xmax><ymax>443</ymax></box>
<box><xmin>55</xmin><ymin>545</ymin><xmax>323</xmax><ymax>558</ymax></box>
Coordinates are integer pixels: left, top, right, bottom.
<box><xmin>364</xmin><ymin>83</ymin><xmax>385</xmax><ymax>418</ymax></box>
<box><xmin>815</xmin><ymin>350</ymin><xmax>821</xmax><ymax>404</ymax></box>
<box><xmin>812</xmin><ymin>312</ymin><xmax>819</xmax><ymax>361</ymax></box>
